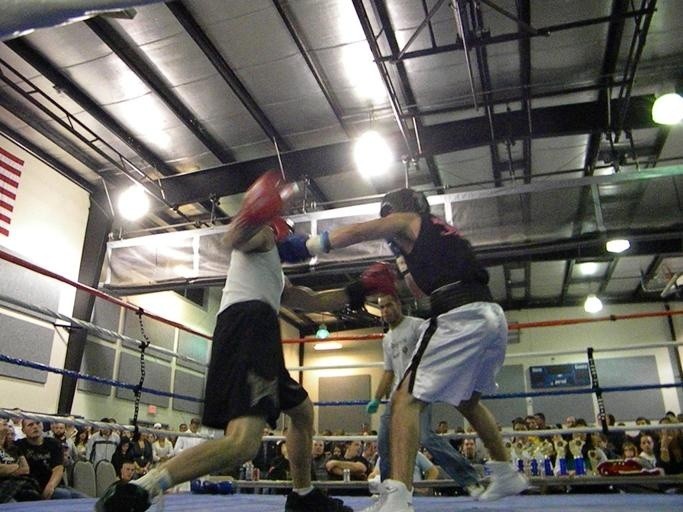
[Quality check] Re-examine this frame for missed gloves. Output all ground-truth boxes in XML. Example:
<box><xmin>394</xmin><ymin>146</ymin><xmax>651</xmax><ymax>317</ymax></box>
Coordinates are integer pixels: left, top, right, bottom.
<box><xmin>366</xmin><ymin>399</ymin><xmax>380</xmax><ymax>414</ymax></box>
<box><xmin>345</xmin><ymin>260</ymin><xmax>396</xmax><ymax>309</ymax></box>
<box><xmin>279</xmin><ymin>231</ymin><xmax>333</xmax><ymax>265</ymax></box>
<box><xmin>237</xmin><ymin>169</ymin><xmax>302</xmax><ymax>229</ymax></box>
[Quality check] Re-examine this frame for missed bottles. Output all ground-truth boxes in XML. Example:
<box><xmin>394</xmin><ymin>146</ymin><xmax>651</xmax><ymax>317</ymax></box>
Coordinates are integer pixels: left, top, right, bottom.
<box><xmin>516</xmin><ymin>456</ymin><xmax>585</xmax><ymax>475</ymax></box>
<box><xmin>343</xmin><ymin>468</ymin><xmax>351</xmax><ymax>482</ymax></box>
<box><xmin>246</xmin><ymin>463</ymin><xmax>260</xmax><ymax>482</ymax></box>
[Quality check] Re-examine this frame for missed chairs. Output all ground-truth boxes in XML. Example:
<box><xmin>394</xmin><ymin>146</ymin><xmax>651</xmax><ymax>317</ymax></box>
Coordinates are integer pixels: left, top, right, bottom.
<box><xmin>93</xmin><ymin>458</ymin><xmax>118</xmax><ymax>498</ymax></box>
<box><xmin>68</xmin><ymin>460</ymin><xmax>97</xmax><ymax>498</ymax></box>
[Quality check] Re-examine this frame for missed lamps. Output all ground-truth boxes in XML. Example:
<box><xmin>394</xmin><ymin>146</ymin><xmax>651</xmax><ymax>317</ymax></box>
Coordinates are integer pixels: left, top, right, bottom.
<box><xmin>651</xmin><ymin>84</ymin><xmax>683</xmax><ymax>126</ymax></box>
<box><xmin>315</xmin><ymin>310</ymin><xmax>329</xmax><ymax>339</ymax></box>
<box><xmin>606</xmin><ymin>239</ymin><xmax>631</xmax><ymax>254</ymax></box>
<box><xmin>582</xmin><ymin>274</ymin><xmax>602</xmax><ymax>314</ymax></box>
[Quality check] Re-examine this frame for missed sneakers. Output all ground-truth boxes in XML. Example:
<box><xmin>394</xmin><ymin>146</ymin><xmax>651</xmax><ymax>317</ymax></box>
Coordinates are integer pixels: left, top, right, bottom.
<box><xmin>284</xmin><ymin>485</ymin><xmax>353</xmax><ymax>511</ymax></box>
<box><xmin>360</xmin><ymin>479</ymin><xmax>416</xmax><ymax>512</ymax></box>
<box><xmin>478</xmin><ymin>459</ymin><xmax>530</xmax><ymax>502</ymax></box>
<box><xmin>466</xmin><ymin>482</ymin><xmax>485</xmax><ymax>498</ymax></box>
<box><xmin>94</xmin><ymin>479</ymin><xmax>152</xmax><ymax>512</ymax></box>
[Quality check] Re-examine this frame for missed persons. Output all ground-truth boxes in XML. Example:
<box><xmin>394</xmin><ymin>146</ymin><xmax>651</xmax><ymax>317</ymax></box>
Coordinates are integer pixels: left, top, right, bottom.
<box><xmin>1</xmin><ymin>407</ymin><xmax>295</xmax><ymax>504</ymax></box>
<box><xmin>91</xmin><ymin>169</ymin><xmax>400</xmax><ymax>512</ymax></box>
<box><xmin>274</xmin><ymin>188</ymin><xmax>532</xmax><ymax>511</ymax></box>
<box><xmin>362</xmin><ymin>288</ymin><xmax>484</xmax><ymax>503</ymax></box>
<box><xmin>311</xmin><ymin>412</ymin><xmax>682</xmax><ymax>497</ymax></box>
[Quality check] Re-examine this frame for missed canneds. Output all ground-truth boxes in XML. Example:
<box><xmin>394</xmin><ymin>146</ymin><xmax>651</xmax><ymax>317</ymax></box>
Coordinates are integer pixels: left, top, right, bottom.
<box><xmin>253</xmin><ymin>468</ymin><xmax>260</xmax><ymax>481</ymax></box>
<box><xmin>343</xmin><ymin>468</ymin><xmax>350</xmax><ymax>483</ymax></box>
